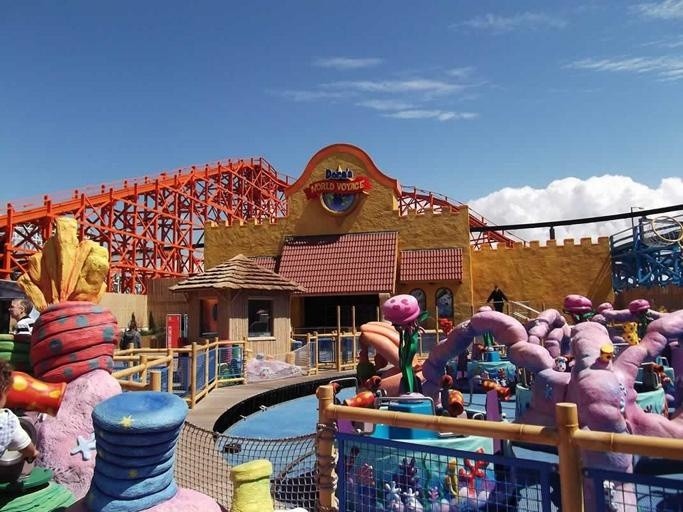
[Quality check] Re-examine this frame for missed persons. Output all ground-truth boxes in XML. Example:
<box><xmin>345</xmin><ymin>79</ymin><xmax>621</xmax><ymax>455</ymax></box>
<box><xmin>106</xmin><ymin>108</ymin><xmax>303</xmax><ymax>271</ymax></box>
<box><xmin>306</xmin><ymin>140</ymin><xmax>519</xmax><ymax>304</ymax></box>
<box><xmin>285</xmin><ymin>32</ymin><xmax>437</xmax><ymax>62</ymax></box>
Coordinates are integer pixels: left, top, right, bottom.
<box><xmin>0</xmin><ymin>360</ymin><xmax>40</xmax><ymax>463</ymax></box>
<box><xmin>486</xmin><ymin>285</ymin><xmax>508</xmax><ymax>313</ymax></box>
<box><xmin>119</xmin><ymin>321</ymin><xmax>142</xmax><ymax>379</ymax></box>
<box><xmin>8</xmin><ymin>298</ymin><xmax>35</xmax><ymax>334</ymax></box>
